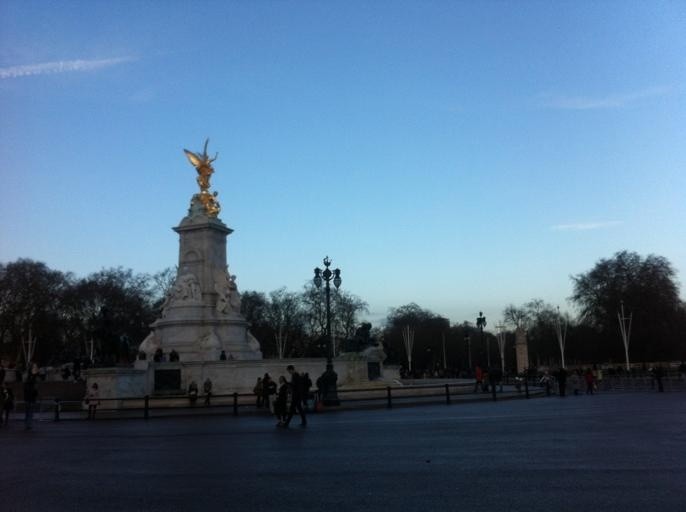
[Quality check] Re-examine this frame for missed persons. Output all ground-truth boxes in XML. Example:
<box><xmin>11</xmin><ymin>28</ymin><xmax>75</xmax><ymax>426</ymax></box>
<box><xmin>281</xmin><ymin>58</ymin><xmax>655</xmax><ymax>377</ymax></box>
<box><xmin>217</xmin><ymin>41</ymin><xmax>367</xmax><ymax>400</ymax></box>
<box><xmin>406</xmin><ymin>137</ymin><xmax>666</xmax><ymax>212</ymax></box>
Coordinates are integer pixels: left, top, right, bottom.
<box><xmin>161</xmin><ymin>264</ymin><xmax>203</xmax><ymax>315</ymax></box>
<box><xmin>0</xmin><ymin>355</ymin><xmax>22</xmax><ymax>430</ymax></box>
<box><xmin>316</xmin><ymin>356</ymin><xmax>334</xmax><ymax>402</ymax></box>
<box><xmin>24</xmin><ymin>361</ymin><xmax>49</xmax><ymax>430</ymax></box>
<box><xmin>187</xmin><ymin>382</ymin><xmax>199</xmax><ymax>405</ymax></box>
<box><xmin>275</xmin><ymin>365</ymin><xmax>309</xmax><ymax>427</ymax></box>
<box><xmin>253</xmin><ymin>376</ymin><xmax>263</xmax><ymax>409</ymax></box>
<box><xmin>261</xmin><ymin>373</ymin><xmax>271</xmax><ymax>407</ymax></box>
<box><xmin>274</xmin><ymin>376</ymin><xmax>289</xmax><ymax>424</ymax></box>
<box><xmin>654</xmin><ymin>366</ymin><xmax>666</xmax><ymax>394</ymax></box>
<box><xmin>214</xmin><ymin>261</ymin><xmax>241</xmax><ymax>315</ymax></box>
<box><xmin>537</xmin><ymin>364</ymin><xmax>605</xmax><ymax>397</ymax></box>
<box><xmin>85</xmin><ymin>382</ymin><xmax>101</xmax><ymax>420</ymax></box>
<box><xmin>303</xmin><ymin>372</ymin><xmax>313</xmax><ymax>405</ymax></box>
<box><xmin>205</xmin><ymin>381</ymin><xmax>214</xmax><ymax>408</ymax></box>
<box><xmin>472</xmin><ymin>363</ymin><xmax>501</xmax><ymax>393</ymax></box>
<box><xmin>218</xmin><ymin>350</ymin><xmax>227</xmax><ymax>361</ymax></box>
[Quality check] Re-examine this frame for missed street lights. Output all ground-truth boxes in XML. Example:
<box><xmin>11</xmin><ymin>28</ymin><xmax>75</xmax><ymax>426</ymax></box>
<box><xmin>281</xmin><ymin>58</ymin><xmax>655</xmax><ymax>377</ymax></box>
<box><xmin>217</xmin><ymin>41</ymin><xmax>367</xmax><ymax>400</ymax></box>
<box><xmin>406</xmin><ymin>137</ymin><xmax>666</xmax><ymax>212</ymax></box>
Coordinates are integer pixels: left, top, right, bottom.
<box><xmin>314</xmin><ymin>256</ymin><xmax>341</xmax><ymax>407</ymax></box>
<box><xmin>477</xmin><ymin>311</ymin><xmax>487</xmax><ymax>373</ymax></box>
